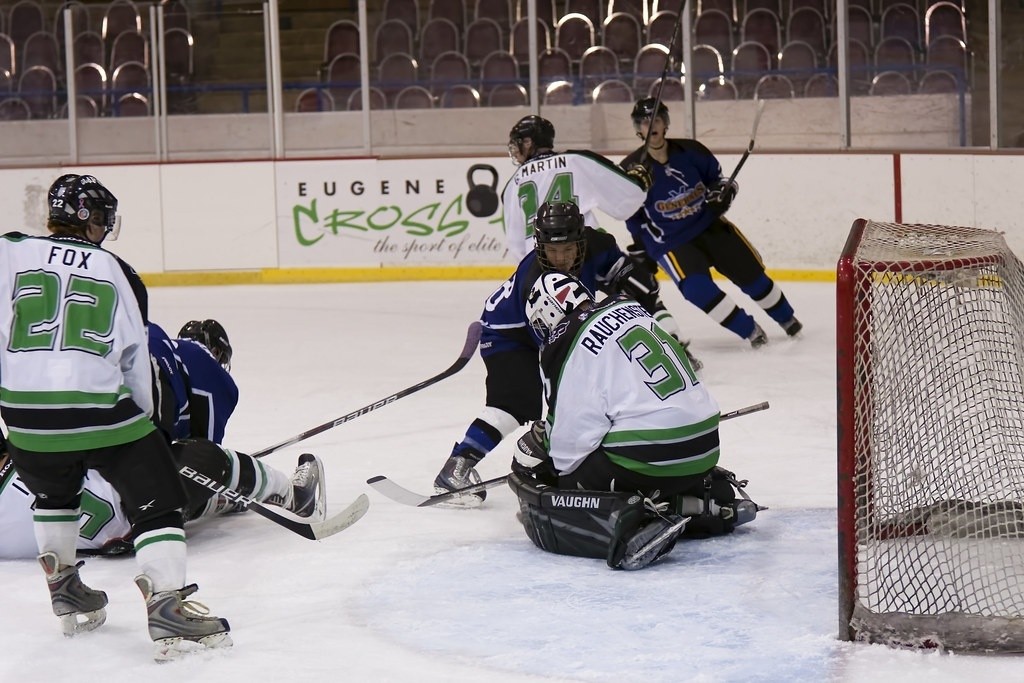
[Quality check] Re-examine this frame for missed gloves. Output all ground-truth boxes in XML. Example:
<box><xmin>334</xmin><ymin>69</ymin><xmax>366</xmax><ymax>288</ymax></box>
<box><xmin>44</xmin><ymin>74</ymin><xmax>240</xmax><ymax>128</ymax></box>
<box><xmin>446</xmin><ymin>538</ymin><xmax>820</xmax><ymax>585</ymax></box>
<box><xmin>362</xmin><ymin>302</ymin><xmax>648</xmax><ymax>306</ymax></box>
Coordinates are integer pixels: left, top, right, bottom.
<box><xmin>627</xmin><ymin>160</ymin><xmax>655</xmax><ymax>191</ymax></box>
<box><xmin>701</xmin><ymin>184</ymin><xmax>737</xmax><ymax>217</ymax></box>
<box><xmin>626</xmin><ymin>244</ymin><xmax>659</xmax><ymax>274</ymax></box>
<box><xmin>512</xmin><ymin>420</ymin><xmax>547</xmax><ymax>477</ymax></box>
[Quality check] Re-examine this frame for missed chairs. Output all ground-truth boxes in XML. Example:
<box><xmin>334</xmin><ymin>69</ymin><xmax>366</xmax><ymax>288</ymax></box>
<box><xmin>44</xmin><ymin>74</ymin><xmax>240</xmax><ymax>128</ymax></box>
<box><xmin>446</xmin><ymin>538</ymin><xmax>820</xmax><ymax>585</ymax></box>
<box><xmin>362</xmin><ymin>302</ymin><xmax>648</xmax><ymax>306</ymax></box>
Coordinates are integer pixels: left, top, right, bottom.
<box><xmin>294</xmin><ymin>0</ymin><xmax>974</xmax><ymax>113</ymax></box>
<box><xmin>0</xmin><ymin>0</ymin><xmax>194</xmax><ymax>120</ymax></box>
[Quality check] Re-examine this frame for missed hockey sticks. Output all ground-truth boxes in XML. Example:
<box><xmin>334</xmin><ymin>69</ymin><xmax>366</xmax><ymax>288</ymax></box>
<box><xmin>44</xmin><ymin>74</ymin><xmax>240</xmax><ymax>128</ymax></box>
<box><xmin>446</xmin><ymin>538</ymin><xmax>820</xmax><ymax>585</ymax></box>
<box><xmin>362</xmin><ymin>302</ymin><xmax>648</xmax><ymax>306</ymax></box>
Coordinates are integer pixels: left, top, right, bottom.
<box><xmin>717</xmin><ymin>99</ymin><xmax>766</xmax><ymax>203</ymax></box>
<box><xmin>366</xmin><ymin>401</ymin><xmax>770</xmax><ymax>508</ymax></box>
<box><xmin>250</xmin><ymin>321</ymin><xmax>483</xmax><ymax>460</ymax></box>
<box><xmin>177</xmin><ymin>462</ymin><xmax>370</xmax><ymax>540</ymax></box>
<box><xmin>640</xmin><ymin>0</ymin><xmax>686</xmax><ymax>164</ymax></box>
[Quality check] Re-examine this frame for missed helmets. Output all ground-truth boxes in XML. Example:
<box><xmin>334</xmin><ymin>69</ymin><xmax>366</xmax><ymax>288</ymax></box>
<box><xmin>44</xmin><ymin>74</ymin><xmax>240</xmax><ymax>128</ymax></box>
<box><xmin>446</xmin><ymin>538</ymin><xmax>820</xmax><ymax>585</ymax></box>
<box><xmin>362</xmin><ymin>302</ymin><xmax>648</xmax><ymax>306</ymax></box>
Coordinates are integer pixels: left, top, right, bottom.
<box><xmin>178</xmin><ymin>319</ymin><xmax>233</xmax><ymax>368</ymax></box>
<box><xmin>525</xmin><ymin>270</ymin><xmax>594</xmax><ymax>341</ymax></box>
<box><xmin>629</xmin><ymin>96</ymin><xmax>668</xmax><ymax>119</ymax></box>
<box><xmin>508</xmin><ymin>116</ymin><xmax>555</xmax><ymax>166</ymax></box>
<box><xmin>534</xmin><ymin>199</ymin><xmax>587</xmax><ymax>276</ymax></box>
<box><xmin>47</xmin><ymin>174</ymin><xmax>117</xmax><ymax>229</ymax></box>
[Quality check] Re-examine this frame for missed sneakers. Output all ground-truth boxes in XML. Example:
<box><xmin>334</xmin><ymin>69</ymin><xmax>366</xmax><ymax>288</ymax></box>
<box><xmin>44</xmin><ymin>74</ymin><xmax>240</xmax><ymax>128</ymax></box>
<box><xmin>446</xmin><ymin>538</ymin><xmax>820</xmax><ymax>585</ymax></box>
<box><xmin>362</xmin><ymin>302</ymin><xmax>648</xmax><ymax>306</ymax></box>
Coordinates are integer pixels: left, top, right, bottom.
<box><xmin>620</xmin><ymin>513</ymin><xmax>693</xmax><ymax>571</ymax></box>
<box><xmin>745</xmin><ymin>323</ymin><xmax>768</xmax><ymax>351</ymax></box>
<box><xmin>433</xmin><ymin>441</ymin><xmax>486</xmax><ymax>509</ymax></box>
<box><xmin>677</xmin><ymin>341</ymin><xmax>702</xmax><ymax>377</ymax></box>
<box><xmin>779</xmin><ymin>316</ymin><xmax>803</xmax><ymax>338</ymax></box>
<box><xmin>37</xmin><ymin>551</ymin><xmax>108</xmax><ymax>638</ymax></box>
<box><xmin>719</xmin><ymin>500</ymin><xmax>757</xmax><ymax>529</ymax></box>
<box><xmin>260</xmin><ymin>453</ymin><xmax>327</xmax><ymax>524</ymax></box>
<box><xmin>132</xmin><ymin>573</ymin><xmax>233</xmax><ymax>664</ymax></box>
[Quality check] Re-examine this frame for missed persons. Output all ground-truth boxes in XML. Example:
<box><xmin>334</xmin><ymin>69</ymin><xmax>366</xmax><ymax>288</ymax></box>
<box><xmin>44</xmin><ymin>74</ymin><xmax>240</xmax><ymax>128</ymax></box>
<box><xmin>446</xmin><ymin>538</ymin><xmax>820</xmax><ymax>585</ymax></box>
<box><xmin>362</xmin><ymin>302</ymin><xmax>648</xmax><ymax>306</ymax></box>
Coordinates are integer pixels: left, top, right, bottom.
<box><xmin>507</xmin><ymin>269</ymin><xmax>757</xmax><ymax>572</ymax></box>
<box><xmin>0</xmin><ymin>174</ymin><xmax>233</xmax><ymax>663</ymax></box>
<box><xmin>502</xmin><ymin>114</ymin><xmax>654</xmax><ymax>266</ymax></box>
<box><xmin>621</xmin><ymin>95</ymin><xmax>802</xmax><ymax>350</ymax></box>
<box><xmin>0</xmin><ymin>320</ymin><xmax>325</xmax><ymax>562</ymax></box>
<box><xmin>435</xmin><ymin>200</ymin><xmax>681</xmax><ymax>509</ymax></box>
